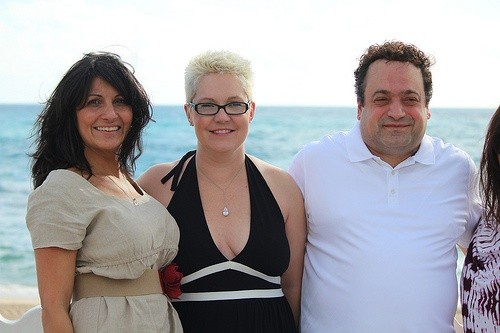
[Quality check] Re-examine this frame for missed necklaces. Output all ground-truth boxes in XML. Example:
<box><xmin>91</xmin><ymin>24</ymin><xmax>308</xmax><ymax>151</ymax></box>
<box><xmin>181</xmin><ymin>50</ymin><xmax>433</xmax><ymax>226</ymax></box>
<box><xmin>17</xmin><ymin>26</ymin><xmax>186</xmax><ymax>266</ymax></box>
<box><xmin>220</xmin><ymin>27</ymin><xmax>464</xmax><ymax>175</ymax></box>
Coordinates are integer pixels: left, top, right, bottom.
<box><xmin>196</xmin><ymin>161</ymin><xmax>246</xmax><ymax>218</ymax></box>
<box><xmin>93</xmin><ymin>166</ymin><xmax>136</xmax><ymax>202</ymax></box>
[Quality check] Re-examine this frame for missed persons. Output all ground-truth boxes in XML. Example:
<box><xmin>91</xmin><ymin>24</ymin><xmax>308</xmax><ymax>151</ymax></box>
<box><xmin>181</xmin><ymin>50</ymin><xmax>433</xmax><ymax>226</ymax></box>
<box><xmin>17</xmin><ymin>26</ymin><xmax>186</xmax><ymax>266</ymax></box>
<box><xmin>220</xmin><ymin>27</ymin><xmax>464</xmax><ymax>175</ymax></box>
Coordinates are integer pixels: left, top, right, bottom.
<box><xmin>288</xmin><ymin>41</ymin><xmax>486</xmax><ymax>333</ymax></box>
<box><xmin>460</xmin><ymin>104</ymin><xmax>500</xmax><ymax>333</ymax></box>
<box><xmin>134</xmin><ymin>52</ymin><xmax>306</xmax><ymax>333</ymax></box>
<box><xmin>25</xmin><ymin>50</ymin><xmax>184</xmax><ymax>332</ymax></box>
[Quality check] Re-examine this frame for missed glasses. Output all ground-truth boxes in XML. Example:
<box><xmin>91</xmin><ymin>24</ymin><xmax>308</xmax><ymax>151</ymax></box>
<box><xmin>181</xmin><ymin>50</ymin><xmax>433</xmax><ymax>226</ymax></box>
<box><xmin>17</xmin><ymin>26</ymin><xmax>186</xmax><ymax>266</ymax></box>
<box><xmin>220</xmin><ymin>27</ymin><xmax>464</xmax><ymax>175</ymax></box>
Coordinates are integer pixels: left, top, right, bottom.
<box><xmin>188</xmin><ymin>100</ymin><xmax>251</xmax><ymax>115</ymax></box>
